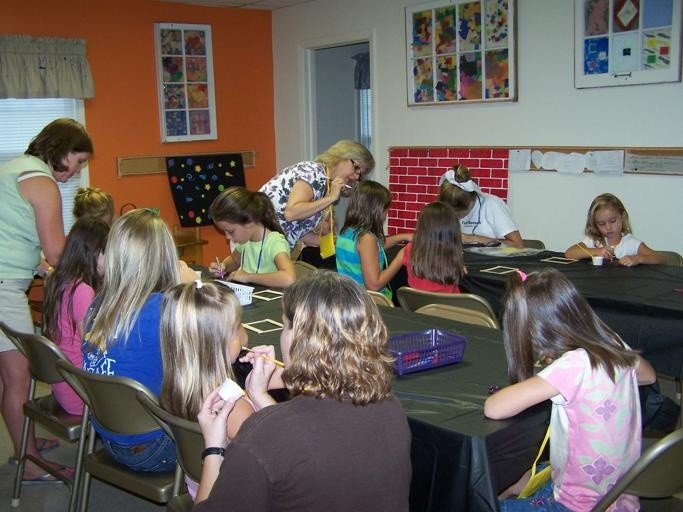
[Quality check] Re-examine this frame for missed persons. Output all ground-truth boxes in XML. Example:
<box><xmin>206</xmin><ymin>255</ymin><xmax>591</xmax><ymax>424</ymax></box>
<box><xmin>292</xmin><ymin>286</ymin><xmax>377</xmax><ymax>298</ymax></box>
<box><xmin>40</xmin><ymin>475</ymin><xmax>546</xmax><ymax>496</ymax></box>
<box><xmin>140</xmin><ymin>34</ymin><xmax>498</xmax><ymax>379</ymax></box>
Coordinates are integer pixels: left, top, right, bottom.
<box><xmin>401</xmin><ymin>199</ymin><xmax>465</xmax><ymax>296</ymax></box>
<box><xmin>206</xmin><ymin>186</ymin><xmax>297</xmax><ymax>290</ymax></box>
<box><xmin>0</xmin><ymin>118</ymin><xmax>94</xmax><ymax>486</ymax></box>
<box><xmin>79</xmin><ymin>208</ymin><xmax>195</xmax><ymax>475</ymax></box>
<box><xmin>481</xmin><ymin>265</ymin><xmax>657</xmax><ymax>512</ymax></box>
<box><xmin>158</xmin><ymin>279</ymin><xmax>287</xmax><ymax>503</ymax></box>
<box><xmin>42</xmin><ymin>215</ymin><xmax>109</xmax><ymax>417</ymax></box>
<box><xmin>436</xmin><ymin>166</ymin><xmax>521</xmax><ymax>246</ymax></box>
<box><xmin>256</xmin><ymin>138</ymin><xmax>374</xmax><ymax>253</ymax></box>
<box><xmin>193</xmin><ymin>270</ymin><xmax>413</xmax><ymax>512</ymax></box>
<box><xmin>73</xmin><ymin>185</ymin><xmax>114</xmax><ymax>225</ymax></box>
<box><xmin>334</xmin><ymin>179</ymin><xmax>414</xmax><ymax>300</ymax></box>
<box><xmin>562</xmin><ymin>193</ymin><xmax>664</xmax><ymax>267</ymax></box>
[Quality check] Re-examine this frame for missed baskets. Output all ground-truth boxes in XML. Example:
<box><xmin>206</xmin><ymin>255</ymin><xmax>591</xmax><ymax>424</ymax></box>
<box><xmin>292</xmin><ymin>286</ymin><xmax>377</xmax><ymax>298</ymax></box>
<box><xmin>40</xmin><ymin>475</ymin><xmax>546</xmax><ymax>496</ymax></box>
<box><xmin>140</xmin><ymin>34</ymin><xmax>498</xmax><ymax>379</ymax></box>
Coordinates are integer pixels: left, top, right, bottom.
<box><xmin>383</xmin><ymin>329</ymin><xmax>468</xmax><ymax>375</ymax></box>
<box><xmin>214</xmin><ymin>279</ymin><xmax>255</xmax><ymax>306</ymax></box>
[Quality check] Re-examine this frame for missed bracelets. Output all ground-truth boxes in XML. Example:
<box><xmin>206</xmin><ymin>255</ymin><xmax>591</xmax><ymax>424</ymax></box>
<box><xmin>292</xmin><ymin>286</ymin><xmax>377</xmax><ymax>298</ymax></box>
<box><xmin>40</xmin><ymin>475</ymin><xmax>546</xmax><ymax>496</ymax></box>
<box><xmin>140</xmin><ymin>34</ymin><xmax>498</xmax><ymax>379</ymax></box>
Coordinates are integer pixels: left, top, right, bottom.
<box><xmin>200</xmin><ymin>446</ymin><xmax>225</xmax><ymax>459</ymax></box>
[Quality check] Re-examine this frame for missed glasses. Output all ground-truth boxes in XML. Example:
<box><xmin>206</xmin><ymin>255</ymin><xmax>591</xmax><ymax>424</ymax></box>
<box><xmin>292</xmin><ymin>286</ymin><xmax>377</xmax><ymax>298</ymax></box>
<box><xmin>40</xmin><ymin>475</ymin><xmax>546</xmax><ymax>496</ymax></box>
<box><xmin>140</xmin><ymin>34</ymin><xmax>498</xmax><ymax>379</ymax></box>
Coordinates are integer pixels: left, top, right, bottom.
<box><xmin>351</xmin><ymin>159</ymin><xmax>363</xmax><ymax>182</ymax></box>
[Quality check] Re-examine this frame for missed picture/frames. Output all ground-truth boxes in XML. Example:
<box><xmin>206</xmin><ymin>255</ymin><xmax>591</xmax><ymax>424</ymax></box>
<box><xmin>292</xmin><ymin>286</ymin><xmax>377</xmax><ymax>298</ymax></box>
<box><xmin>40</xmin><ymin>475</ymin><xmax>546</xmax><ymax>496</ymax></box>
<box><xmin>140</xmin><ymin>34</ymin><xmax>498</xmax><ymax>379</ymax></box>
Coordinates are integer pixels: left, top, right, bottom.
<box><xmin>154</xmin><ymin>22</ymin><xmax>218</xmax><ymax>143</ymax></box>
<box><xmin>573</xmin><ymin>0</ymin><xmax>683</xmax><ymax>89</ymax></box>
<box><xmin>405</xmin><ymin>0</ymin><xmax>518</xmax><ymax>107</ymax></box>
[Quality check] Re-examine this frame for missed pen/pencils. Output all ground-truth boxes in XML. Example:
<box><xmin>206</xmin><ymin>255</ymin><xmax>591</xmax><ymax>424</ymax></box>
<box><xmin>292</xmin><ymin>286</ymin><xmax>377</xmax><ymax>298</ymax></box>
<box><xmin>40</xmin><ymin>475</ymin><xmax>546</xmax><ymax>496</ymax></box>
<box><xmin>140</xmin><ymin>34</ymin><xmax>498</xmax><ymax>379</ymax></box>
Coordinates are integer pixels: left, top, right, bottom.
<box><xmin>215</xmin><ymin>256</ymin><xmax>224</xmax><ymax>279</ymax></box>
<box><xmin>605</xmin><ymin>237</ymin><xmax>613</xmax><ymax>262</ymax></box>
<box><xmin>536</xmin><ymin>355</ymin><xmax>546</xmax><ymax>364</ymax></box>
<box><xmin>241</xmin><ymin>346</ymin><xmax>288</xmax><ymax>369</ymax></box>
<box><xmin>575</xmin><ymin>242</ymin><xmax>593</xmax><ymax>258</ymax></box>
<box><xmin>322</xmin><ymin>175</ymin><xmax>353</xmax><ymax>189</ymax></box>
<box><xmin>407</xmin><ymin>356</ymin><xmax>434</xmax><ymax>369</ymax></box>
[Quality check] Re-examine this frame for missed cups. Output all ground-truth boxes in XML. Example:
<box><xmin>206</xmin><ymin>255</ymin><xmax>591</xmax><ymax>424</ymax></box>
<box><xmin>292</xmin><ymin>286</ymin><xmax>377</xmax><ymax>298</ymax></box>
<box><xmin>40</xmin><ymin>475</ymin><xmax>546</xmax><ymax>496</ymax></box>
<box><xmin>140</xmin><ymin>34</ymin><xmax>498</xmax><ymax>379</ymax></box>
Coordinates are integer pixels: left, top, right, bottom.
<box><xmin>591</xmin><ymin>256</ymin><xmax>604</xmax><ymax>267</ymax></box>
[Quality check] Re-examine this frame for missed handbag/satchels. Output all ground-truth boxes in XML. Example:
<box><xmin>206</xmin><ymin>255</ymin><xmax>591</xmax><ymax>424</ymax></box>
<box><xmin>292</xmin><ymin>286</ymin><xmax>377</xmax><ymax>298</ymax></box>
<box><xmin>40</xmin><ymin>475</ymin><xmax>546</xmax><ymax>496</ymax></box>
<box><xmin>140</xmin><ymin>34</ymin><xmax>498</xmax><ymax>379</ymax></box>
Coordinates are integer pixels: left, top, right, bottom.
<box><xmin>516</xmin><ymin>464</ymin><xmax>552</xmax><ymax>498</ymax></box>
<box><xmin>319</xmin><ymin>232</ymin><xmax>335</xmax><ymax>259</ymax></box>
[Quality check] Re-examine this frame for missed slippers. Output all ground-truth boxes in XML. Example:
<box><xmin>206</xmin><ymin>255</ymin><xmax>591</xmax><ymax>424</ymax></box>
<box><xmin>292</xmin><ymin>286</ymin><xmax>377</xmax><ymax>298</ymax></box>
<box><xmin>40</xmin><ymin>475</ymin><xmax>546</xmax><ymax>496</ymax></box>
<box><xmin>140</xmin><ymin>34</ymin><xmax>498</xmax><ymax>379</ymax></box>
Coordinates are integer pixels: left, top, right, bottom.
<box><xmin>9</xmin><ymin>439</ymin><xmax>76</xmax><ymax>484</ymax></box>
